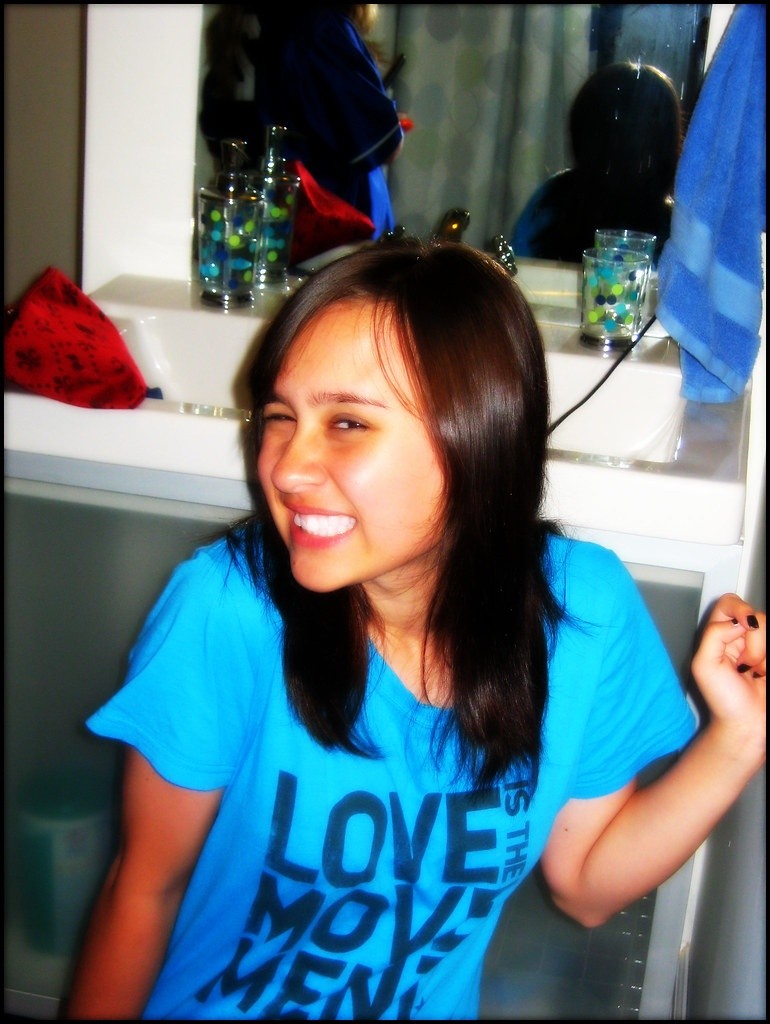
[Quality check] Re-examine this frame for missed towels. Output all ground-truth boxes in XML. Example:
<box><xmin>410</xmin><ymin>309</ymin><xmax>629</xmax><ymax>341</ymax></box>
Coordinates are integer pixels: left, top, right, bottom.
<box><xmin>658</xmin><ymin>0</ymin><xmax>770</xmax><ymax>401</ymax></box>
<box><xmin>282</xmin><ymin>162</ymin><xmax>375</xmax><ymax>258</ymax></box>
<box><xmin>1</xmin><ymin>264</ymin><xmax>147</xmax><ymax>410</ymax></box>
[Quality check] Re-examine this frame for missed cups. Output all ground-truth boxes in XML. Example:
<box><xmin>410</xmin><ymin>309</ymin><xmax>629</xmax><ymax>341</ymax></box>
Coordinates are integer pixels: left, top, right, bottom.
<box><xmin>595</xmin><ymin>229</ymin><xmax>658</xmax><ymax>334</ymax></box>
<box><xmin>580</xmin><ymin>246</ymin><xmax>649</xmax><ymax>353</ymax></box>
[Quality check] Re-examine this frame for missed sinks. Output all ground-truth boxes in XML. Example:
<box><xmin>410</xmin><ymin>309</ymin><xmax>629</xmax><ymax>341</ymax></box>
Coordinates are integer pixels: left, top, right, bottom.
<box><xmin>109</xmin><ymin>311</ymin><xmax>690</xmax><ymax>468</ymax></box>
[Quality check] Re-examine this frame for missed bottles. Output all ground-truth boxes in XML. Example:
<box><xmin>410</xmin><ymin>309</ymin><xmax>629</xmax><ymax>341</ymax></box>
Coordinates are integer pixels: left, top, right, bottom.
<box><xmin>190</xmin><ymin>138</ymin><xmax>265</xmax><ymax>311</ymax></box>
<box><xmin>238</xmin><ymin>123</ymin><xmax>302</xmax><ymax>293</ymax></box>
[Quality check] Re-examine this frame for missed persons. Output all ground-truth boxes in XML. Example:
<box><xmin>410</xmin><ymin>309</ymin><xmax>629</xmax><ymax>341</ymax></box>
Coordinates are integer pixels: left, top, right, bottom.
<box><xmin>54</xmin><ymin>236</ymin><xmax>766</xmax><ymax>1020</ymax></box>
<box><xmin>511</xmin><ymin>62</ymin><xmax>685</xmax><ymax>262</ymax></box>
<box><xmin>200</xmin><ymin>2</ymin><xmax>414</xmax><ymax>273</ymax></box>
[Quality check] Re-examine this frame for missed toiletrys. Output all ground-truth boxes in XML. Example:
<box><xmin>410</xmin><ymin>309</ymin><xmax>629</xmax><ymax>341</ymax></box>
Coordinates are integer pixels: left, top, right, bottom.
<box><xmin>217</xmin><ymin>123</ymin><xmax>300</xmax><ymax>297</ymax></box>
<box><xmin>200</xmin><ymin>137</ymin><xmax>260</xmax><ymax>311</ymax></box>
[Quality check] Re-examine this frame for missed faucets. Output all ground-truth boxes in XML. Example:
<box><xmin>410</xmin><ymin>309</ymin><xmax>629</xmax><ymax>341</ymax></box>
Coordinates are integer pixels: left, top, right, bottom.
<box><xmin>434</xmin><ymin>204</ymin><xmax>520</xmax><ymax>276</ymax></box>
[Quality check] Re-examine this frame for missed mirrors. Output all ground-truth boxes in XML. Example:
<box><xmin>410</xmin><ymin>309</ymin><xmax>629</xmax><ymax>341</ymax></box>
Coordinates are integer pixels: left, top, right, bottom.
<box><xmin>81</xmin><ymin>0</ymin><xmax>733</xmax><ymax>467</ymax></box>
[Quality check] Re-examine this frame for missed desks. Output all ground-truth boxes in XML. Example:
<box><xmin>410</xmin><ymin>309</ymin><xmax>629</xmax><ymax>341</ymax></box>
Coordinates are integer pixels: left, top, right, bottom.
<box><xmin>2</xmin><ymin>390</ymin><xmax>765</xmax><ymax>1020</ymax></box>
